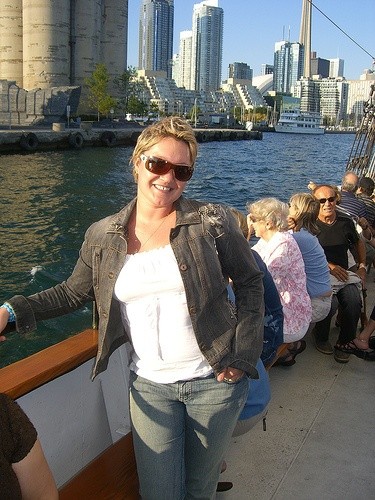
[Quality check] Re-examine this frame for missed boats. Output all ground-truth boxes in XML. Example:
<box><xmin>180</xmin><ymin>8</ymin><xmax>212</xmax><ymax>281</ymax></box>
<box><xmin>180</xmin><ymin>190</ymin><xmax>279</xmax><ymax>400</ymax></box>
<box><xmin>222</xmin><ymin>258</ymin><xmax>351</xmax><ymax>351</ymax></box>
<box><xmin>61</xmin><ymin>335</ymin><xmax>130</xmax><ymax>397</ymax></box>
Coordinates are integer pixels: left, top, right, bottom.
<box><xmin>273</xmin><ymin>109</ymin><xmax>324</xmax><ymax>135</ymax></box>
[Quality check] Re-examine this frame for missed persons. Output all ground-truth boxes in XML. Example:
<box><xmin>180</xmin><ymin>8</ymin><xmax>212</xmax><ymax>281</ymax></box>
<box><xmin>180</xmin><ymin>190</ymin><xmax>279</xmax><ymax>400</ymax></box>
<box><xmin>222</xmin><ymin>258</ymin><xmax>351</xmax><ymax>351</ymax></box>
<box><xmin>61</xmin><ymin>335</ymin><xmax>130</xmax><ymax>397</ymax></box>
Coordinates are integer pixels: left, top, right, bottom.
<box><xmin>0</xmin><ymin>393</ymin><xmax>59</xmax><ymax>500</ymax></box>
<box><xmin>246</xmin><ymin>197</ymin><xmax>312</xmax><ymax>366</ymax></box>
<box><xmin>0</xmin><ymin>117</ymin><xmax>265</xmax><ymax>500</ymax></box>
<box><xmin>308</xmin><ymin>172</ymin><xmax>375</xmax><ymax>363</ymax></box>
<box><xmin>216</xmin><ymin>283</ymin><xmax>272</xmax><ymax>492</ymax></box>
<box><xmin>215</xmin><ymin>207</ymin><xmax>287</xmax><ymax>372</ymax></box>
<box><xmin>287</xmin><ymin>193</ymin><xmax>332</xmax><ymax>333</ymax></box>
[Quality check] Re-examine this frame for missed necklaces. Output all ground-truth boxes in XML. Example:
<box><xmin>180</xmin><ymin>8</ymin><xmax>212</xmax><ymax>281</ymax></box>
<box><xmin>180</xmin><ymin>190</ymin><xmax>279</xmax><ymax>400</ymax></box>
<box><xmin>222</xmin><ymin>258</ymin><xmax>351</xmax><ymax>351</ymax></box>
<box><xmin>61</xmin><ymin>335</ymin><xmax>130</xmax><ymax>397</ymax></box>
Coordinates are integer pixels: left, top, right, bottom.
<box><xmin>132</xmin><ymin>207</ymin><xmax>173</xmax><ymax>253</ymax></box>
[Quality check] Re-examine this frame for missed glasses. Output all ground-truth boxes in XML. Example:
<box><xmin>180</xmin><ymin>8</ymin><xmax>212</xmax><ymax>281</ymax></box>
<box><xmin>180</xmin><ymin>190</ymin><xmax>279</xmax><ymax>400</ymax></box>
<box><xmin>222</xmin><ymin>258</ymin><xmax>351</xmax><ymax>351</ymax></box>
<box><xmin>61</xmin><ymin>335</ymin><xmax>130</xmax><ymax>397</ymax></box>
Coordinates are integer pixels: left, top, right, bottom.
<box><xmin>319</xmin><ymin>196</ymin><xmax>336</xmax><ymax>203</ymax></box>
<box><xmin>141</xmin><ymin>154</ymin><xmax>195</xmax><ymax>184</ymax></box>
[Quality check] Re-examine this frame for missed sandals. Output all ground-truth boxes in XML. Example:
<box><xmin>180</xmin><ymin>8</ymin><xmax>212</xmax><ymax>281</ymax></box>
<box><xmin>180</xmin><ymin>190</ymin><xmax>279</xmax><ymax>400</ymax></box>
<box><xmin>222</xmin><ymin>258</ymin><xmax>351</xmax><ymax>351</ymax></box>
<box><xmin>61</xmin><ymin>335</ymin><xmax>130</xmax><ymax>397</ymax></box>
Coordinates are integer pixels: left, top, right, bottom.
<box><xmin>287</xmin><ymin>340</ymin><xmax>306</xmax><ymax>354</ymax></box>
<box><xmin>336</xmin><ymin>336</ymin><xmax>369</xmax><ymax>351</ymax></box>
<box><xmin>279</xmin><ymin>352</ymin><xmax>296</xmax><ymax>366</ymax></box>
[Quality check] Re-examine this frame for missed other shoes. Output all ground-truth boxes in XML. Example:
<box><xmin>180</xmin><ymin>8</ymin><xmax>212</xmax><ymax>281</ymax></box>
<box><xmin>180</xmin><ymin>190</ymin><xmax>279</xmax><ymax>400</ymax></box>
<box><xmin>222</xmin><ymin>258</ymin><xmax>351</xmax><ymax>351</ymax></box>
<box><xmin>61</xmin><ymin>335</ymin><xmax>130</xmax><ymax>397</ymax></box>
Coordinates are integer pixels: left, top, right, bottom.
<box><xmin>217</xmin><ymin>482</ymin><xmax>233</xmax><ymax>492</ymax></box>
<box><xmin>311</xmin><ymin>333</ymin><xmax>333</xmax><ymax>354</ymax></box>
<box><xmin>335</xmin><ymin>343</ymin><xmax>351</xmax><ymax>361</ymax></box>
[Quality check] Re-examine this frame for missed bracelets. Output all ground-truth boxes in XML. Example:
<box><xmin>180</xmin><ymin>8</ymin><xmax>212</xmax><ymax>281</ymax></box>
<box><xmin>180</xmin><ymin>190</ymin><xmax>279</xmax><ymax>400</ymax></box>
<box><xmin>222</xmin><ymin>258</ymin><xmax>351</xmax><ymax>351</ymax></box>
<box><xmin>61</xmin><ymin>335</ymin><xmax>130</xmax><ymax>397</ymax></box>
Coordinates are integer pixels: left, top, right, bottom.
<box><xmin>362</xmin><ymin>225</ymin><xmax>368</xmax><ymax>230</ymax></box>
<box><xmin>1</xmin><ymin>303</ymin><xmax>15</xmax><ymax>322</ymax></box>
<box><xmin>359</xmin><ymin>263</ymin><xmax>367</xmax><ymax>272</ymax></box>
